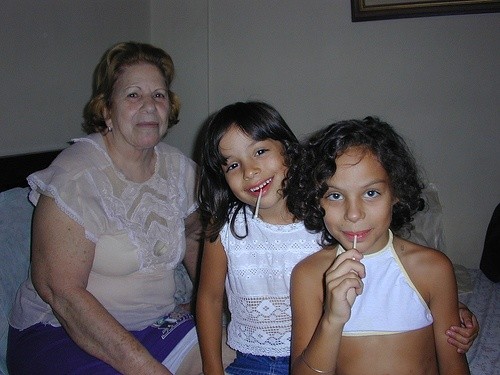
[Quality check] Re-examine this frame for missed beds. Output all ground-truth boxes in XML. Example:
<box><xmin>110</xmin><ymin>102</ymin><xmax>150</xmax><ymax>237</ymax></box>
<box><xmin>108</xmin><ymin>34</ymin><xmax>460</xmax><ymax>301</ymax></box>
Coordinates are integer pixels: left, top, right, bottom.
<box><xmin>0</xmin><ymin>146</ymin><xmax>500</xmax><ymax>374</ymax></box>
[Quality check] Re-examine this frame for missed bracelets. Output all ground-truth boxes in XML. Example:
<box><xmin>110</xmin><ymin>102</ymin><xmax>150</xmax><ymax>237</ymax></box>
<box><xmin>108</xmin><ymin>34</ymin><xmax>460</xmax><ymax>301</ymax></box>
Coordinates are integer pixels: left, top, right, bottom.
<box><xmin>302</xmin><ymin>349</ymin><xmax>334</xmax><ymax>375</ymax></box>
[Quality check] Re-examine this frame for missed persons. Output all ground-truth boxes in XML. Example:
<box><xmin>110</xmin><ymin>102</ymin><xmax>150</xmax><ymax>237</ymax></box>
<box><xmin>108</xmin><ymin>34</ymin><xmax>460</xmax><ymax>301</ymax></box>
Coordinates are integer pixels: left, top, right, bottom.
<box><xmin>196</xmin><ymin>100</ymin><xmax>479</xmax><ymax>375</ymax></box>
<box><xmin>280</xmin><ymin>116</ymin><xmax>471</xmax><ymax>375</ymax></box>
<box><xmin>7</xmin><ymin>43</ymin><xmax>237</xmax><ymax>375</ymax></box>
